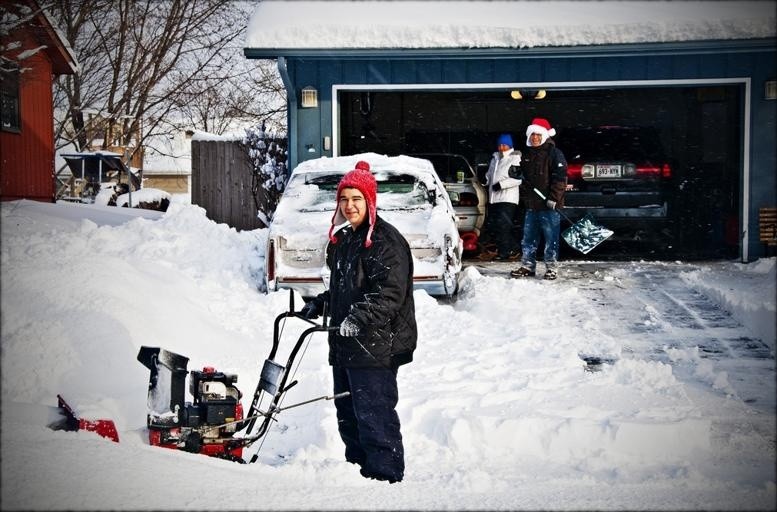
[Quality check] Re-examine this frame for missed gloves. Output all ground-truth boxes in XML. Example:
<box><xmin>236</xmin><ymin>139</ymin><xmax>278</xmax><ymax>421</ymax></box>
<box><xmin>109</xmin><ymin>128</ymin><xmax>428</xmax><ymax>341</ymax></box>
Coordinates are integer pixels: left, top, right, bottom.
<box><xmin>492</xmin><ymin>182</ymin><xmax>501</xmax><ymax>191</ymax></box>
<box><xmin>509</xmin><ymin>166</ymin><xmax>519</xmax><ymax>177</ymax></box>
<box><xmin>339</xmin><ymin>315</ymin><xmax>362</xmax><ymax>336</ymax></box>
<box><xmin>547</xmin><ymin>199</ymin><xmax>556</xmax><ymax>210</ymax></box>
<box><xmin>477</xmin><ymin>173</ymin><xmax>488</xmax><ymax>184</ymax></box>
<box><xmin>300</xmin><ymin>301</ymin><xmax>324</xmax><ymax>318</ymax></box>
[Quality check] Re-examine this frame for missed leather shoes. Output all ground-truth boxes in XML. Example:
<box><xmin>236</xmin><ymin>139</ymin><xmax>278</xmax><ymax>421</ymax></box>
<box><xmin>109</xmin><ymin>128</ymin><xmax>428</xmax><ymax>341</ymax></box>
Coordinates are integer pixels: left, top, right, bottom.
<box><xmin>494</xmin><ymin>248</ymin><xmax>521</xmax><ymax>260</ymax></box>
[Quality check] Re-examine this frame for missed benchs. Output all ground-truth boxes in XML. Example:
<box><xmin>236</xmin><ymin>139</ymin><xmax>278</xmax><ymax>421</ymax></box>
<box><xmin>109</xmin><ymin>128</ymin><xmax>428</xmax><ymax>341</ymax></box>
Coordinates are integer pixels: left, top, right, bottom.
<box><xmin>758</xmin><ymin>207</ymin><xmax>777</xmax><ymax>257</ymax></box>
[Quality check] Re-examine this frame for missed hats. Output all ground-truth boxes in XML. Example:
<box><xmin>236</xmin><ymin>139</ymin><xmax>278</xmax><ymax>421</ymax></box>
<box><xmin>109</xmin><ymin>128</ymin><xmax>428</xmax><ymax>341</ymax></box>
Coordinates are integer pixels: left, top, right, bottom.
<box><xmin>526</xmin><ymin>119</ymin><xmax>555</xmax><ymax>146</ymax></box>
<box><xmin>495</xmin><ymin>134</ymin><xmax>513</xmax><ymax>148</ymax></box>
<box><xmin>331</xmin><ymin>162</ymin><xmax>377</xmax><ymax>225</ymax></box>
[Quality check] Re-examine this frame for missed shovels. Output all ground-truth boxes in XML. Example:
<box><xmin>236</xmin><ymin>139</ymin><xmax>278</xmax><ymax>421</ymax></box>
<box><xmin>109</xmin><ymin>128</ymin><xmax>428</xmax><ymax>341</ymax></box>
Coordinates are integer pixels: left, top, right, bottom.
<box><xmin>512</xmin><ymin>167</ymin><xmax>615</xmax><ymax>255</ymax></box>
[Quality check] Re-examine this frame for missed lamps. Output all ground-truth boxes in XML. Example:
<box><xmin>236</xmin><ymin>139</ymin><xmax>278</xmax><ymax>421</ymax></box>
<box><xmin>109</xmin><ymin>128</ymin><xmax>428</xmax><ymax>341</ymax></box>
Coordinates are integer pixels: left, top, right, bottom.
<box><xmin>764</xmin><ymin>77</ymin><xmax>777</xmax><ymax>101</ymax></box>
<box><xmin>302</xmin><ymin>85</ymin><xmax>318</xmax><ymax>108</ymax></box>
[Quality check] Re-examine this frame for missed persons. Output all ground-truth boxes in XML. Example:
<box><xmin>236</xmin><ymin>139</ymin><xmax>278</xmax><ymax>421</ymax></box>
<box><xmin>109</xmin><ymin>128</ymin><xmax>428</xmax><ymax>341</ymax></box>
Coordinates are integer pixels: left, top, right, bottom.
<box><xmin>474</xmin><ymin>132</ymin><xmax>523</xmax><ymax>263</ymax></box>
<box><xmin>506</xmin><ymin>115</ymin><xmax>568</xmax><ymax>282</ymax></box>
<box><xmin>295</xmin><ymin>160</ymin><xmax>418</xmax><ymax>485</ymax></box>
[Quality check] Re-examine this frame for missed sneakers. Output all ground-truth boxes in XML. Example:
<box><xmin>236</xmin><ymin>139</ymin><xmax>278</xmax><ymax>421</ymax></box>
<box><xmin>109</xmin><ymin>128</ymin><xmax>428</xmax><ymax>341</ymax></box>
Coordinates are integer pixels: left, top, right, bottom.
<box><xmin>545</xmin><ymin>270</ymin><xmax>557</xmax><ymax>278</ymax></box>
<box><xmin>511</xmin><ymin>267</ymin><xmax>535</xmax><ymax>277</ymax></box>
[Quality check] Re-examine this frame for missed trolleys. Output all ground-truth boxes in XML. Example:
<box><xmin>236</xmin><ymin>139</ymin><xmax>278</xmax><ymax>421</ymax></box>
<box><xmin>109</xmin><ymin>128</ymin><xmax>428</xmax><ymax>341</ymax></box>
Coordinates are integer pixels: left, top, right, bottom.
<box><xmin>49</xmin><ymin>286</ymin><xmax>356</xmax><ymax>467</ymax></box>
